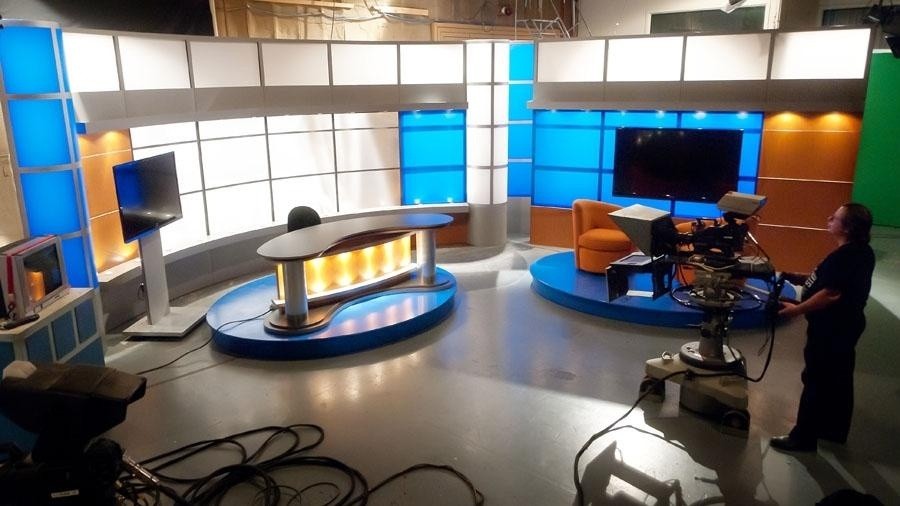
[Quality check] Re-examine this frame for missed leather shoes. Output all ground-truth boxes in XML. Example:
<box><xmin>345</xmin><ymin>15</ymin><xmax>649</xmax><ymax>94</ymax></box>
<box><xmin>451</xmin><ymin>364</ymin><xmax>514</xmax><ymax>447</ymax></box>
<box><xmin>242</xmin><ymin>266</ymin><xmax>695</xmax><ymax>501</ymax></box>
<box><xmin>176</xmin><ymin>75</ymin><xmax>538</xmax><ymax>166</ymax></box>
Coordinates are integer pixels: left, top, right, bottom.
<box><xmin>819</xmin><ymin>426</ymin><xmax>852</xmax><ymax>443</ymax></box>
<box><xmin>771</xmin><ymin>432</ymin><xmax>821</xmax><ymax>454</ymax></box>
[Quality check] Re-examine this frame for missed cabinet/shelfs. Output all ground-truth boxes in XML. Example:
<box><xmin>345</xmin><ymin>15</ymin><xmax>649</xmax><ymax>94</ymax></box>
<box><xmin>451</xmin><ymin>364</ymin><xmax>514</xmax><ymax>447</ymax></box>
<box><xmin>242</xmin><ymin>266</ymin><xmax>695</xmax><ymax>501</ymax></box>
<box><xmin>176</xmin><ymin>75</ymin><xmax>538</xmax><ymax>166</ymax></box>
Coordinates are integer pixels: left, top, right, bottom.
<box><xmin>0</xmin><ymin>286</ymin><xmax>108</xmax><ymax>370</ymax></box>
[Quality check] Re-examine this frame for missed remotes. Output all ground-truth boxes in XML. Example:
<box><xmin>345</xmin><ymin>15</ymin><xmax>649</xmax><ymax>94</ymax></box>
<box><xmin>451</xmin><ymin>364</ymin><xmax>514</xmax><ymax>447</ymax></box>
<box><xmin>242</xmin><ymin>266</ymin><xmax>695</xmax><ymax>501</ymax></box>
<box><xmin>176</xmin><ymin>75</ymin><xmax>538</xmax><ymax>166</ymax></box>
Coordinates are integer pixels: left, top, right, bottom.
<box><xmin>2</xmin><ymin>313</ymin><xmax>41</xmax><ymax>331</ymax></box>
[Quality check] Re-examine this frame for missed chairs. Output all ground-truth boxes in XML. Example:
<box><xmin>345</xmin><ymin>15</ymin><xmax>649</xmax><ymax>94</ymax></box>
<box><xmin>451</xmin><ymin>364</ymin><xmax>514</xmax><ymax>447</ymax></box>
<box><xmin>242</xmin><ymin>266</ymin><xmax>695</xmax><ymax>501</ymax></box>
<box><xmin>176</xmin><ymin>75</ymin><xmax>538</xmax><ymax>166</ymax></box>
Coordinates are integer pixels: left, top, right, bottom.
<box><xmin>285</xmin><ymin>206</ymin><xmax>321</xmax><ymax>233</ymax></box>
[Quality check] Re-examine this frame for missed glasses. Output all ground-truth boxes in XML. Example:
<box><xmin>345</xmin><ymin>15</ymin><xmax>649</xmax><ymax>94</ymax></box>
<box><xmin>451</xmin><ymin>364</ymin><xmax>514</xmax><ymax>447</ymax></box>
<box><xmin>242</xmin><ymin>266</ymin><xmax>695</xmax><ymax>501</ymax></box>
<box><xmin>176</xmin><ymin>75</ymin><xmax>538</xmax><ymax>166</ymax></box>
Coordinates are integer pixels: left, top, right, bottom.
<box><xmin>829</xmin><ymin>215</ymin><xmax>844</xmax><ymax>222</ymax></box>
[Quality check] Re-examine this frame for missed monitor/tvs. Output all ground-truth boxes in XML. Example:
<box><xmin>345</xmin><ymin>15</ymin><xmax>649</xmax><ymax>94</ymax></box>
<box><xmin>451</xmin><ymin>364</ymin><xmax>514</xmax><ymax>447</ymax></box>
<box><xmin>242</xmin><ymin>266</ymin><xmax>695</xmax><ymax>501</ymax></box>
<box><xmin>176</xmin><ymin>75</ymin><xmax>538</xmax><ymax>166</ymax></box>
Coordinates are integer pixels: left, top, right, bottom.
<box><xmin>107</xmin><ymin>150</ymin><xmax>184</xmax><ymax>243</ymax></box>
<box><xmin>610</xmin><ymin>123</ymin><xmax>743</xmax><ymax>205</ymax></box>
<box><xmin>2</xmin><ymin>234</ymin><xmax>72</xmax><ymax>316</ymax></box>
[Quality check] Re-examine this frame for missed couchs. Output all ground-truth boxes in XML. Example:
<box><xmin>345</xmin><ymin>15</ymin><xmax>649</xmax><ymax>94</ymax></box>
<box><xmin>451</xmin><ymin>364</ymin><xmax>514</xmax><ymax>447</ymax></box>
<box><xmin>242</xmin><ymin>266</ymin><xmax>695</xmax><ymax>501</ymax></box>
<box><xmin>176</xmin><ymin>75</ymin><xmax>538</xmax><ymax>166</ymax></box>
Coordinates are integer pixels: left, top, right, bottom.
<box><xmin>572</xmin><ymin>198</ymin><xmax>641</xmax><ymax>274</ymax></box>
<box><xmin>671</xmin><ymin>216</ymin><xmax>766</xmax><ymax>292</ymax></box>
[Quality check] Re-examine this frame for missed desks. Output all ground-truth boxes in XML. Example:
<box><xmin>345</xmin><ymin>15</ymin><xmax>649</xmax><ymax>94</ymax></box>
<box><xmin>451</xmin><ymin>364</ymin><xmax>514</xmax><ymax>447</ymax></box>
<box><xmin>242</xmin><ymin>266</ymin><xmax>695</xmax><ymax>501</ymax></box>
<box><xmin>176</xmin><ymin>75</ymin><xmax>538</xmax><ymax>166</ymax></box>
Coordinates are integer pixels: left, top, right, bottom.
<box><xmin>255</xmin><ymin>211</ymin><xmax>454</xmax><ymax>337</ymax></box>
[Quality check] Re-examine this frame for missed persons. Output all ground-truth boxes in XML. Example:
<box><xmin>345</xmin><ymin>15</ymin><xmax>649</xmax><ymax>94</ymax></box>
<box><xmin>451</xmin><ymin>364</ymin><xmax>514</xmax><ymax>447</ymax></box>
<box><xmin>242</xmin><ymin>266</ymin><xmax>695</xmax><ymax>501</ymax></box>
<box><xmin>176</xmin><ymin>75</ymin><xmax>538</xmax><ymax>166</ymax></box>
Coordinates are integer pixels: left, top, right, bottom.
<box><xmin>769</xmin><ymin>200</ymin><xmax>877</xmax><ymax>454</ymax></box>
